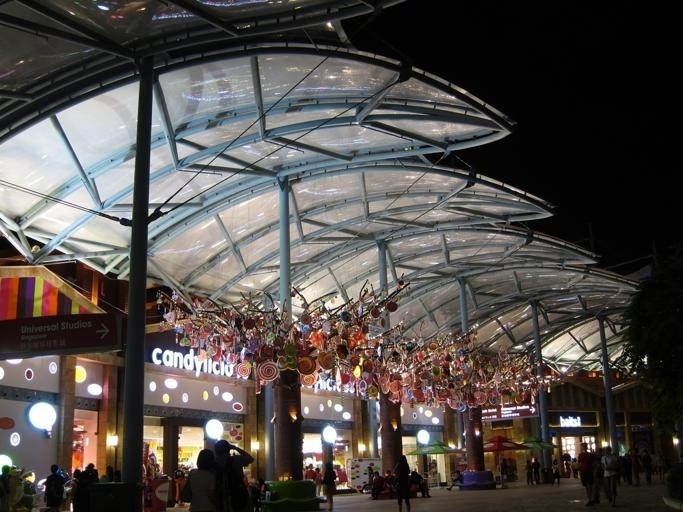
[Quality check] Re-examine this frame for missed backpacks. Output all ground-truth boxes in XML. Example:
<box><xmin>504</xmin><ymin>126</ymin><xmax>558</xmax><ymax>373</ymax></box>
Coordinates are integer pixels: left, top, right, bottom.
<box><xmin>212</xmin><ymin>459</ymin><xmax>248</xmax><ymax>512</ymax></box>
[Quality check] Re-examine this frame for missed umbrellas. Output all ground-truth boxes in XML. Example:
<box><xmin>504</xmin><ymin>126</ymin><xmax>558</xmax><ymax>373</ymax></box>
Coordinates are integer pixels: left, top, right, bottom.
<box><xmin>519</xmin><ymin>437</ymin><xmax>558</xmax><ymax>459</ymax></box>
<box><xmin>406</xmin><ymin>440</ymin><xmax>467</xmax><ymax>473</ymax></box>
<box><xmin>482</xmin><ymin>436</ymin><xmax>533</xmax><ymax>484</ymax></box>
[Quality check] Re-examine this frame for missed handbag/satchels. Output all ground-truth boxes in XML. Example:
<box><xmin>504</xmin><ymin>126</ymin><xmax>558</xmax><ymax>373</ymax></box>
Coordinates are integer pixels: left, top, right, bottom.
<box><xmin>178</xmin><ymin>478</ymin><xmax>191</xmax><ymax>502</ymax></box>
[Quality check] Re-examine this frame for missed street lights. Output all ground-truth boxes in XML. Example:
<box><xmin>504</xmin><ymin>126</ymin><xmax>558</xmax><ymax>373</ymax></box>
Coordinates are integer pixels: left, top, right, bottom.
<box><xmin>417</xmin><ymin>429</ymin><xmax>431</xmax><ymax>497</ymax></box>
<box><xmin>322</xmin><ymin>423</ymin><xmax>340</xmax><ymax>512</ymax></box>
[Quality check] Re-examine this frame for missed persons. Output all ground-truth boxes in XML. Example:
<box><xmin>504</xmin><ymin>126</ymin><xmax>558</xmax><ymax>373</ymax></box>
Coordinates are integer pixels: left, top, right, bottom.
<box><xmin>303</xmin><ymin>464</ymin><xmax>323</xmax><ymax>496</ymax></box>
<box><xmin>371</xmin><ymin>470</ymin><xmax>432</xmax><ymax>500</ymax></box>
<box><xmin>0</xmin><ymin>462</ymin><xmax>121</xmax><ymax>512</ymax></box>
<box><xmin>394</xmin><ymin>454</ymin><xmax>413</xmax><ymax>512</ymax></box>
<box><xmin>500</xmin><ymin>457</ymin><xmax>518</xmax><ymax>482</ymax></box>
<box><xmin>565</xmin><ymin>458</ymin><xmax>577</xmax><ymax>478</ymax></box>
<box><xmin>525</xmin><ymin>457</ymin><xmax>561</xmax><ymax>487</ymax></box>
<box><xmin>323</xmin><ymin>462</ymin><xmax>338</xmax><ymax>512</ymax></box>
<box><xmin>577</xmin><ymin>443</ymin><xmax>666</xmax><ymax>506</ymax></box>
<box><xmin>179</xmin><ymin>439</ymin><xmax>255</xmax><ymax>512</ymax></box>
<box><xmin>446</xmin><ymin>470</ymin><xmax>464</xmax><ymax>490</ymax></box>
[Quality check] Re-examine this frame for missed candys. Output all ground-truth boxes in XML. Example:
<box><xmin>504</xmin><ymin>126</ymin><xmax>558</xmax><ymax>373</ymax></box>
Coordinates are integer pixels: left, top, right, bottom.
<box><xmin>159</xmin><ymin>280</ymin><xmax>537</xmax><ymax>412</ymax></box>
